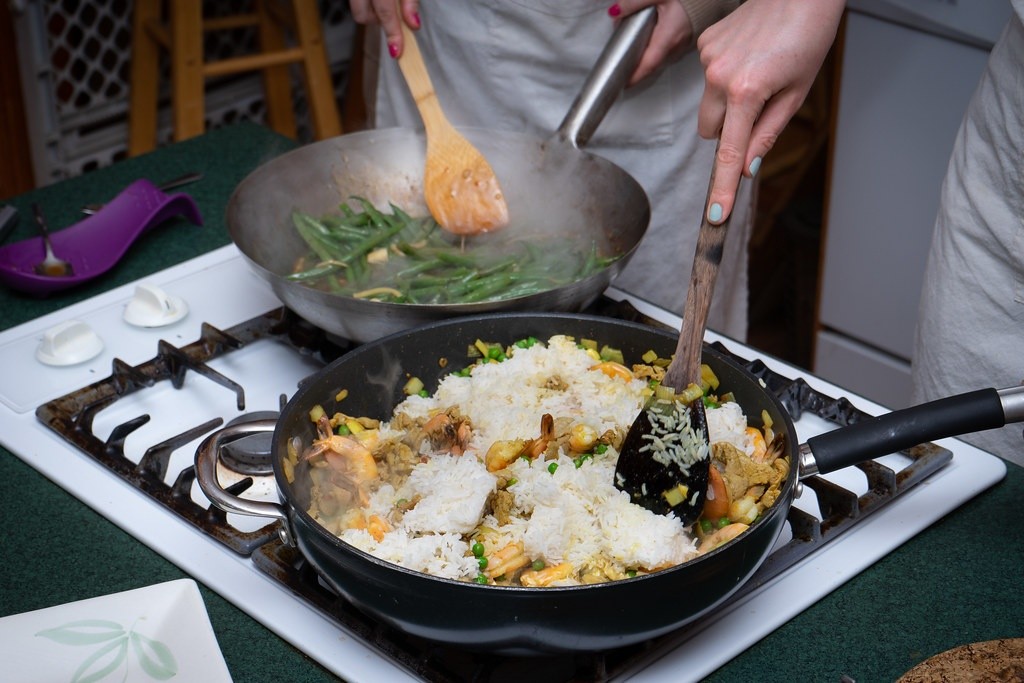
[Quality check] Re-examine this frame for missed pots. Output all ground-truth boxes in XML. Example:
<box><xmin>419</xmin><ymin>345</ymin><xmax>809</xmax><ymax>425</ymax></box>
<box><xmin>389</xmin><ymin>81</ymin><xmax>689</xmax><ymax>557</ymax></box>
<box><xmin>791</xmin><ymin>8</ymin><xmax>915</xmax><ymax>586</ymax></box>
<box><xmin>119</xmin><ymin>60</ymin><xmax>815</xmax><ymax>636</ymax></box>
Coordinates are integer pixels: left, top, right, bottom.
<box><xmin>225</xmin><ymin>5</ymin><xmax>659</xmax><ymax>351</ymax></box>
<box><xmin>191</xmin><ymin>308</ymin><xmax>1024</xmax><ymax>660</ymax></box>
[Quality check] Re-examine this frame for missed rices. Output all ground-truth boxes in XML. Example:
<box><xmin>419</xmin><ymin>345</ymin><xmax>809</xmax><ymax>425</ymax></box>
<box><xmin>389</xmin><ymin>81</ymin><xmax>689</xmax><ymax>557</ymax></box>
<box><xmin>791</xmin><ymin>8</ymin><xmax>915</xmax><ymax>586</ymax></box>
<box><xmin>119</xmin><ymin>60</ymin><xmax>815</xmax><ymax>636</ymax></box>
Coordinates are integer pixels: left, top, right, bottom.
<box><xmin>285</xmin><ymin>331</ymin><xmax>789</xmax><ymax>592</ymax></box>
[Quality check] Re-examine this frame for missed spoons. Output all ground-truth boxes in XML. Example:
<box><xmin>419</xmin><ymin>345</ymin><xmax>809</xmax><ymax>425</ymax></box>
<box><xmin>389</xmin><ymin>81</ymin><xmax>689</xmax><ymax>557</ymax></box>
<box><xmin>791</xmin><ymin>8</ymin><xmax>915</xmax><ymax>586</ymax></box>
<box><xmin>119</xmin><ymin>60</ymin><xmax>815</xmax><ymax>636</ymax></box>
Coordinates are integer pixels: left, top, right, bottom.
<box><xmin>27</xmin><ymin>200</ymin><xmax>73</xmax><ymax>281</ymax></box>
<box><xmin>80</xmin><ymin>170</ymin><xmax>208</xmax><ymax>218</ymax></box>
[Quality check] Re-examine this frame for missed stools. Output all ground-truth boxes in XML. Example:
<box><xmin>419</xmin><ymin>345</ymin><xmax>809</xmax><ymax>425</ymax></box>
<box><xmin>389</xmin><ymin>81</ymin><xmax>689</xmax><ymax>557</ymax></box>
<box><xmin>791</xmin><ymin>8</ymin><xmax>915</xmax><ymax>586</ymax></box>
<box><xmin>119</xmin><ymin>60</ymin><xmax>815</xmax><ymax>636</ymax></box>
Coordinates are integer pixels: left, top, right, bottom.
<box><xmin>130</xmin><ymin>0</ymin><xmax>346</xmax><ymax>141</ymax></box>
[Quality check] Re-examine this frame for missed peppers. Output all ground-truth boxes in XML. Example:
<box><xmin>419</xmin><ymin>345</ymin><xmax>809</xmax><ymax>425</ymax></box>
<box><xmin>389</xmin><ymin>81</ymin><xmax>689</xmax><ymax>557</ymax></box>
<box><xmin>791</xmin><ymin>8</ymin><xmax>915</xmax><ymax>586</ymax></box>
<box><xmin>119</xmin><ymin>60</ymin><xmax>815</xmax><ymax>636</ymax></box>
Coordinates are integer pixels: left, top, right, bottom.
<box><xmin>285</xmin><ymin>195</ymin><xmax>624</xmax><ymax>307</ymax></box>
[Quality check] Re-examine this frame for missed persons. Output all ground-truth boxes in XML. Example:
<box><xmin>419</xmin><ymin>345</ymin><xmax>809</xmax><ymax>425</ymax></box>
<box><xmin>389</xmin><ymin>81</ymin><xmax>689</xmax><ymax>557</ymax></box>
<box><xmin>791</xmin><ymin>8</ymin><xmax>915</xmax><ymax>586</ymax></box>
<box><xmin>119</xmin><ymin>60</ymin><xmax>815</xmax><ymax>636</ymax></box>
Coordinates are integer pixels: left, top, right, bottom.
<box><xmin>344</xmin><ymin>0</ymin><xmax>751</xmax><ymax>345</ymax></box>
<box><xmin>690</xmin><ymin>0</ymin><xmax>1024</xmax><ymax>468</ymax></box>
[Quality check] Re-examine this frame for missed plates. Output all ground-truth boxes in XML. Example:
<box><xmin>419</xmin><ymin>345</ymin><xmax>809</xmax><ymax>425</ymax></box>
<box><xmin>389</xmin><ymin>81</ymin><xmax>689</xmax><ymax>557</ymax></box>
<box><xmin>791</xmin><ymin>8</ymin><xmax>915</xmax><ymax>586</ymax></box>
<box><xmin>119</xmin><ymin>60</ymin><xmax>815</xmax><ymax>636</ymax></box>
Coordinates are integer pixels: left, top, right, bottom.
<box><xmin>0</xmin><ymin>575</ymin><xmax>235</xmax><ymax>683</ymax></box>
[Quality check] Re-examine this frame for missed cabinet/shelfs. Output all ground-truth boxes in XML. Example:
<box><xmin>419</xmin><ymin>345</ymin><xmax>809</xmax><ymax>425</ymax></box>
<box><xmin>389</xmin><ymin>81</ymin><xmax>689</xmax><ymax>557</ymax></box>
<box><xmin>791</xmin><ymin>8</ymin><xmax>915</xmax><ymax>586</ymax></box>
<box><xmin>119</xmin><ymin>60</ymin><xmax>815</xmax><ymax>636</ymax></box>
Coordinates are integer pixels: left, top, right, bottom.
<box><xmin>809</xmin><ymin>0</ymin><xmax>1015</xmax><ymax>412</ymax></box>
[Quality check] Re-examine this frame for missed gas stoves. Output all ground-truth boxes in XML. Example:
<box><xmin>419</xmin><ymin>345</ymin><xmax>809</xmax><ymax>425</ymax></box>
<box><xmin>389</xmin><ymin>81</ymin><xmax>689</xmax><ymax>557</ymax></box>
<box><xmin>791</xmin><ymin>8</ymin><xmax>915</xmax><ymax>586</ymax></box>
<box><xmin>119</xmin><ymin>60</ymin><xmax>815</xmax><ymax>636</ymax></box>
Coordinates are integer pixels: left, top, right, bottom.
<box><xmin>0</xmin><ymin>244</ymin><xmax>1009</xmax><ymax>683</ymax></box>
